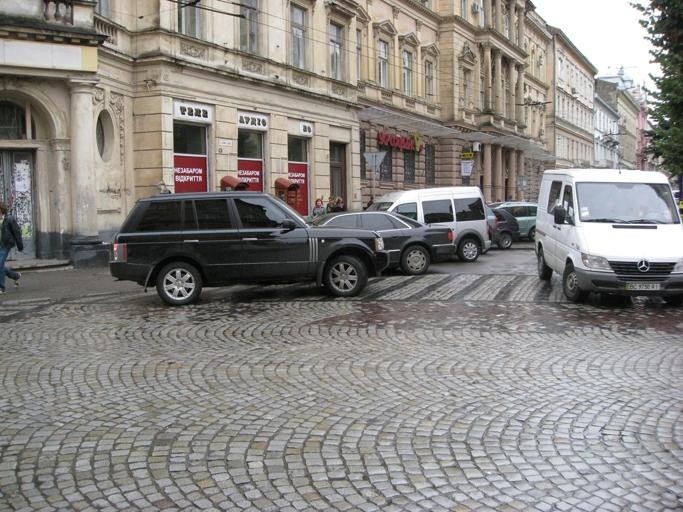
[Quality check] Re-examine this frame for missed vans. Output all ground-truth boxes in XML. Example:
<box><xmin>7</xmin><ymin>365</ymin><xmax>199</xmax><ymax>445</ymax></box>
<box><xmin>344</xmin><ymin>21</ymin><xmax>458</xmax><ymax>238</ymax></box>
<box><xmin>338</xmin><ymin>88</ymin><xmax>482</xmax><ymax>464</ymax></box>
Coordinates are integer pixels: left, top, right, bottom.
<box><xmin>535</xmin><ymin>168</ymin><xmax>683</xmax><ymax>305</ymax></box>
<box><xmin>364</xmin><ymin>185</ymin><xmax>492</xmax><ymax>262</ymax></box>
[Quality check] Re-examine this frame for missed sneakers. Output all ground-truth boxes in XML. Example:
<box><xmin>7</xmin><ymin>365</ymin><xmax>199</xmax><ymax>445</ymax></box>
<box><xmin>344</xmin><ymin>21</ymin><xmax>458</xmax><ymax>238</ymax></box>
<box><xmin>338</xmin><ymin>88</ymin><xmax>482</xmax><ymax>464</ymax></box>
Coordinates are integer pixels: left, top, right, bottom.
<box><xmin>14</xmin><ymin>272</ymin><xmax>21</xmax><ymax>287</ymax></box>
<box><xmin>1</xmin><ymin>288</ymin><xmax>8</xmax><ymax>294</ymax></box>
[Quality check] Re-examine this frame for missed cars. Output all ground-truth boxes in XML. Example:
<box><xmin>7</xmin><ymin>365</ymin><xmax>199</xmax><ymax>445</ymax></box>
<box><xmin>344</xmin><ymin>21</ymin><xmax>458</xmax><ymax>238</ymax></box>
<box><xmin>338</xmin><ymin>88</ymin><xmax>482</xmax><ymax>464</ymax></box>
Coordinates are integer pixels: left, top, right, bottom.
<box><xmin>307</xmin><ymin>210</ymin><xmax>456</xmax><ymax>276</ymax></box>
<box><xmin>490</xmin><ymin>208</ymin><xmax>520</xmax><ymax>249</ymax></box>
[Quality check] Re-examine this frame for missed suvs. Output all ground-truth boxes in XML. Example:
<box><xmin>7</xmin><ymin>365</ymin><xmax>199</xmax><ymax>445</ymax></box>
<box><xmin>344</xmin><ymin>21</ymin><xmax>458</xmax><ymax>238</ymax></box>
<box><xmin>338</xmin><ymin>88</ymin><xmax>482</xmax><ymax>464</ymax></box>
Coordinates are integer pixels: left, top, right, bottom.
<box><xmin>487</xmin><ymin>200</ymin><xmax>537</xmax><ymax>242</ymax></box>
<box><xmin>108</xmin><ymin>191</ymin><xmax>378</xmax><ymax>306</ymax></box>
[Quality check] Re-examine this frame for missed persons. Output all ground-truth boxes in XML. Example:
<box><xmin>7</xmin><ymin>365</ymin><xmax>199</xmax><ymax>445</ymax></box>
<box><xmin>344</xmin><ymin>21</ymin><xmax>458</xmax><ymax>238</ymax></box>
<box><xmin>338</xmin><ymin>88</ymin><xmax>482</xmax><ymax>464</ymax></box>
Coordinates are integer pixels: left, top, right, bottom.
<box><xmin>0</xmin><ymin>201</ymin><xmax>23</xmax><ymax>295</ymax></box>
<box><xmin>311</xmin><ymin>196</ymin><xmax>345</xmax><ymax>221</ymax></box>
